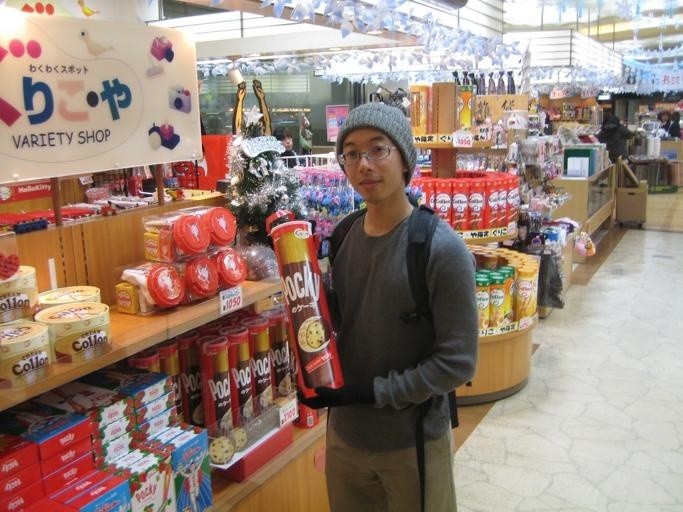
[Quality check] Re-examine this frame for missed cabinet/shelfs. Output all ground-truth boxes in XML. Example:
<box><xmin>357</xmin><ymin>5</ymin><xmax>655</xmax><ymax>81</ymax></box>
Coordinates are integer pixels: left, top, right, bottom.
<box><xmin>404</xmin><ymin>80</ymin><xmax>535</xmax><ymax>406</ymax></box>
<box><xmin>0</xmin><ymin>189</ymin><xmax>327</xmax><ymax>512</ymax></box>
<box><xmin>549</xmin><ymin>162</ymin><xmax>619</xmax><ymax>240</ymax></box>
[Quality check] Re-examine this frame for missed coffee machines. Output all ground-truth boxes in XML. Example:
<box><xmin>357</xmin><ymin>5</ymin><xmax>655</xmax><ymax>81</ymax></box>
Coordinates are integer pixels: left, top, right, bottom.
<box><xmin>630</xmin><ymin>134</ymin><xmax>645</xmax><ymax>157</ymax></box>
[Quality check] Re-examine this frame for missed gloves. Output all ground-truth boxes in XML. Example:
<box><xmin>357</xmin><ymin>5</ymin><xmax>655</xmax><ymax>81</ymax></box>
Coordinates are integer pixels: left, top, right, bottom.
<box><xmin>296</xmin><ymin>382</ymin><xmax>374</xmax><ymax>409</ymax></box>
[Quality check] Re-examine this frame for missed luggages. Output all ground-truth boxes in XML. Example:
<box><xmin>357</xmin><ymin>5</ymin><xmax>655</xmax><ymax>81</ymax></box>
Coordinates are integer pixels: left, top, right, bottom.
<box><xmin>615</xmin><ymin>155</ymin><xmax>648</xmax><ymax>229</ymax></box>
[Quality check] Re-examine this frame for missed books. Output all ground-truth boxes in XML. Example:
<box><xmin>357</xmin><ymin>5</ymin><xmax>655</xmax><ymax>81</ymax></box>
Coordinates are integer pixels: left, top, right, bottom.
<box><xmin>562</xmin><ymin>141</ymin><xmax>607</xmax><ymax>178</ymax></box>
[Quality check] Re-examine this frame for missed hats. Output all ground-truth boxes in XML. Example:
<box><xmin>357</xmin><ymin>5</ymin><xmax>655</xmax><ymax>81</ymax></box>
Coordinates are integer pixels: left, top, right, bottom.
<box><xmin>336</xmin><ymin>101</ymin><xmax>416</xmax><ymax>187</ymax></box>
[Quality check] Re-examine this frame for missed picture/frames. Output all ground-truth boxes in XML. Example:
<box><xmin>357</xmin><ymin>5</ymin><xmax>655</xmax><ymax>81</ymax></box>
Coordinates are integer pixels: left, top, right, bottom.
<box><xmin>324</xmin><ymin>104</ymin><xmax>349</xmax><ymax>143</ymax></box>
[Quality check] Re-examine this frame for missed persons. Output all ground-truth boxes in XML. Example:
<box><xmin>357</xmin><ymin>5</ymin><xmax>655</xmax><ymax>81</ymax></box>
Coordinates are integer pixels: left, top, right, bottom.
<box><xmin>273</xmin><ymin>126</ymin><xmax>300</xmax><ymax>169</ymax></box>
<box><xmin>290</xmin><ymin>103</ymin><xmax>479</xmax><ymax>512</ymax></box>
<box><xmin>598</xmin><ymin>116</ymin><xmax>637</xmax><ymax>186</ymax></box>
<box><xmin>657</xmin><ymin>110</ymin><xmax>670</xmax><ymax>136</ymax></box>
<box><xmin>667</xmin><ymin>112</ymin><xmax>682</xmax><ymax>137</ymax></box>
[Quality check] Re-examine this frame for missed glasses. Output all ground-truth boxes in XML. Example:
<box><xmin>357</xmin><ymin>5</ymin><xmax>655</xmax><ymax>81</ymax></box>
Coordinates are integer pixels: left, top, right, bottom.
<box><xmin>337</xmin><ymin>145</ymin><xmax>399</xmax><ymax>166</ymax></box>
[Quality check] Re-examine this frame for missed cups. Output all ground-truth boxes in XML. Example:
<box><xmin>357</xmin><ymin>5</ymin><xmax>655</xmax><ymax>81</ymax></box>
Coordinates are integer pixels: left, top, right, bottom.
<box><xmin>645</xmin><ymin>134</ymin><xmax>661</xmax><ymax>160</ymax></box>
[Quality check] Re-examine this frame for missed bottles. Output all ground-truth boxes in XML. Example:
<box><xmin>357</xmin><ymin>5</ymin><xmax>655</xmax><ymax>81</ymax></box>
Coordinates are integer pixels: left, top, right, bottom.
<box><xmin>549</xmin><ymin>85</ymin><xmax>595</xmax><ymax>121</ymax></box>
<box><xmin>92</xmin><ymin>168</ymin><xmax>127</xmax><ymax>196</ymax></box>
<box><xmin>456</xmin><ymin>111</ymin><xmax>539</xmax><ymax>177</ymax></box>
